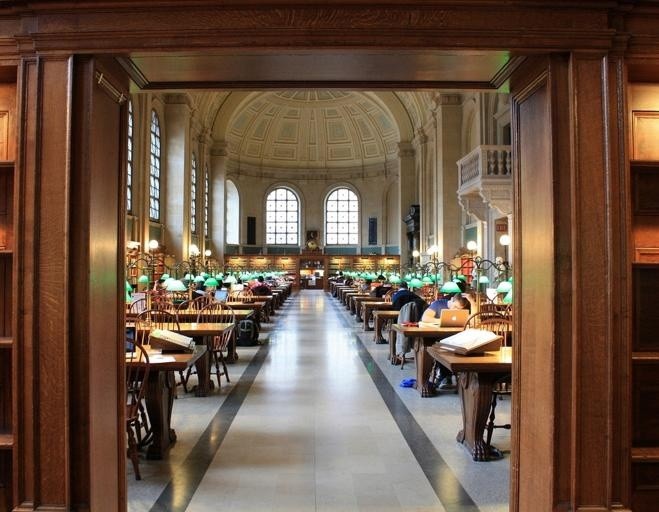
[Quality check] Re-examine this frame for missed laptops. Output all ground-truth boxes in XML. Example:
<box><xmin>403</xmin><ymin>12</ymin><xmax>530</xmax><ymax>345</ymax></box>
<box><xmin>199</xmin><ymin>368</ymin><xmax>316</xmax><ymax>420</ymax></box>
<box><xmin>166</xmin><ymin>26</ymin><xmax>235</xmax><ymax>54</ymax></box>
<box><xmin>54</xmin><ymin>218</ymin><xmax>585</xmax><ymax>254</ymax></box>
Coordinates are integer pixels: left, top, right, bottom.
<box><xmin>432</xmin><ymin>309</ymin><xmax>469</xmax><ymax>327</ymax></box>
<box><xmin>126</xmin><ymin>327</ymin><xmax>137</xmax><ymax>359</ymax></box>
<box><xmin>214</xmin><ymin>290</ymin><xmax>229</xmax><ymax>302</ymax></box>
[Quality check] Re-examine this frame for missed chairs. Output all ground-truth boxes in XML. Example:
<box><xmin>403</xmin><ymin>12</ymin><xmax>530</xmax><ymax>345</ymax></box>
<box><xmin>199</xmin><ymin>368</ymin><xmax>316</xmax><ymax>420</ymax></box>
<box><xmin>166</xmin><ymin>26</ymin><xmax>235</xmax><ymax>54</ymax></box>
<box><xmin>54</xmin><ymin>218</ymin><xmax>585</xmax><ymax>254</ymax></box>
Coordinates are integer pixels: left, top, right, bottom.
<box><xmin>124</xmin><ymin>289</ymin><xmax>253</xmax><ymax>481</ymax></box>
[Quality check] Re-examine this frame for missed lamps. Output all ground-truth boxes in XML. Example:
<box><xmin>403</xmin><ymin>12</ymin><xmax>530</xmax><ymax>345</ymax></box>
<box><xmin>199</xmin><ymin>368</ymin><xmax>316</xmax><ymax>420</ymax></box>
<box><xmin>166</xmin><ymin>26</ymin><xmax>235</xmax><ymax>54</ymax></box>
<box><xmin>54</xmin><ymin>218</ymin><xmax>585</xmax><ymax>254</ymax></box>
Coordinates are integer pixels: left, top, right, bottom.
<box><xmin>409</xmin><ymin>255</ymin><xmax>513</xmax><ymax>303</ymax></box>
<box><xmin>125</xmin><ymin>252</ymin><xmax>225</xmax><ymax>302</ymax></box>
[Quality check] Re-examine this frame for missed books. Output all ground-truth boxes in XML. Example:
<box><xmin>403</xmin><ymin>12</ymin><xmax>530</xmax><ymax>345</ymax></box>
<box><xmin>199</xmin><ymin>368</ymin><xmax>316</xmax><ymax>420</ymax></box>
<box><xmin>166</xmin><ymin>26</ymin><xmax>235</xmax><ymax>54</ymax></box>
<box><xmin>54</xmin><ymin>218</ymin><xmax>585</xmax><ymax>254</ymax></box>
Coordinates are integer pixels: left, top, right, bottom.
<box><xmin>148</xmin><ymin>329</ymin><xmax>193</xmax><ymax>351</ymax></box>
<box><xmin>161</xmin><ymin>343</ymin><xmax>197</xmax><ymax>354</ymax></box>
<box><xmin>431</xmin><ymin>341</ymin><xmax>501</xmax><ymax>352</ymax></box>
<box><xmin>439</xmin><ymin>329</ymin><xmax>505</xmax><ymax>356</ymax></box>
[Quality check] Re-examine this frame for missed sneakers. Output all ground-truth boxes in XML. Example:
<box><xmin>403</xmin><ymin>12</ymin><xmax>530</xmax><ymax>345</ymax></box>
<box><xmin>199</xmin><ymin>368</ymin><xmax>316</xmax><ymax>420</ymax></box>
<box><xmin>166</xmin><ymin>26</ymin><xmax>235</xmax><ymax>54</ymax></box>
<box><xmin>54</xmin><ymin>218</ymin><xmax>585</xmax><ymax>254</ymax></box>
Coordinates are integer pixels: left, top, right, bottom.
<box><xmin>439</xmin><ymin>383</ymin><xmax>452</xmax><ymax>390</ymax></box>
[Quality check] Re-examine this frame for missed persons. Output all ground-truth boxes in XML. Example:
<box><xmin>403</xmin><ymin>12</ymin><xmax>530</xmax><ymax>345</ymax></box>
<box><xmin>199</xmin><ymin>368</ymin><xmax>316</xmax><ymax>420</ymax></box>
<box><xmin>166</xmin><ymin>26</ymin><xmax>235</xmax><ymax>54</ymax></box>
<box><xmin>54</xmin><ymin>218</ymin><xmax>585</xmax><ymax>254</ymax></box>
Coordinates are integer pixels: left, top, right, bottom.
<box><xmin>332</xmin><ymin>271</ymin><xmax>479</xmax><ymax>390</ymax></box>
<box><xmin>154</xmin><ymin>274</ymin><xmax>279</xmax><ymax>309</ymax></box>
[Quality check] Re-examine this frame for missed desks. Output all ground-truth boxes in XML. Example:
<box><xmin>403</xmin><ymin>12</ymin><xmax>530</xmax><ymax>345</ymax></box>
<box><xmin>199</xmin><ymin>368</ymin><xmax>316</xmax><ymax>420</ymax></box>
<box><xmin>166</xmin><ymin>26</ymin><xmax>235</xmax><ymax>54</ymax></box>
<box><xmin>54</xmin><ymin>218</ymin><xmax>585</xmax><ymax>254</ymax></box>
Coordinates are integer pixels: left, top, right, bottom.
<box><xmin>335</xmin><ymin>285</ymin><xmax>513</xmax><ymax>462</ymax></box>
<box><xmin>124</xmin><ymin>289</ymin><xmax>284</xmax><ymax>317</ymax></box>
